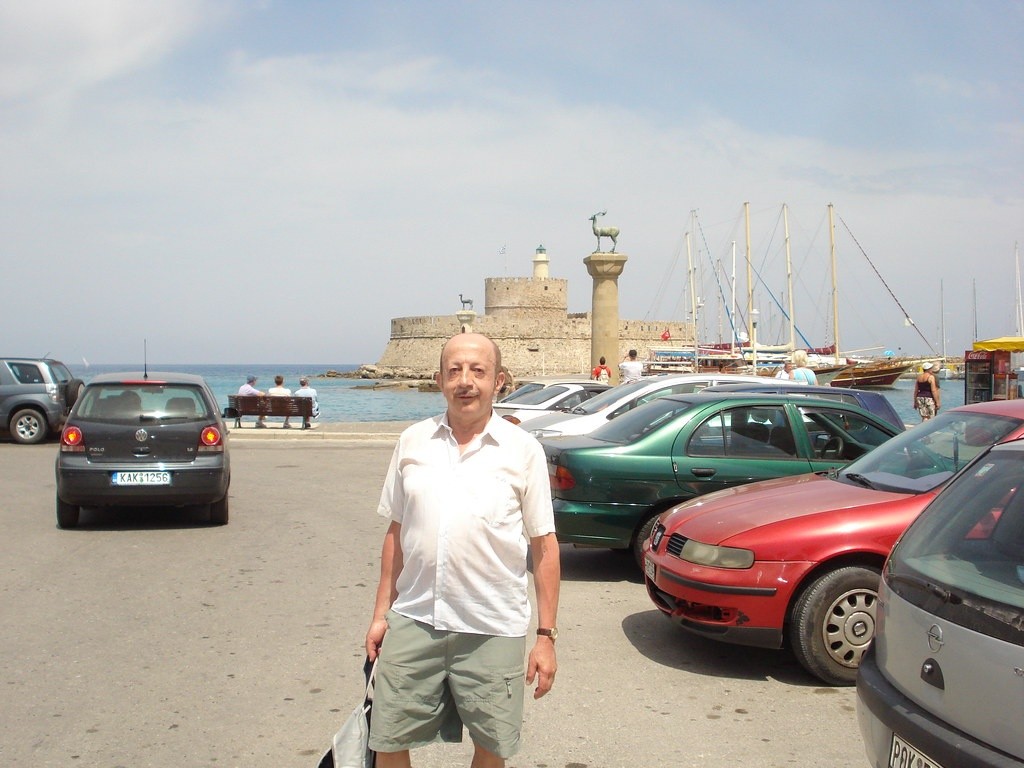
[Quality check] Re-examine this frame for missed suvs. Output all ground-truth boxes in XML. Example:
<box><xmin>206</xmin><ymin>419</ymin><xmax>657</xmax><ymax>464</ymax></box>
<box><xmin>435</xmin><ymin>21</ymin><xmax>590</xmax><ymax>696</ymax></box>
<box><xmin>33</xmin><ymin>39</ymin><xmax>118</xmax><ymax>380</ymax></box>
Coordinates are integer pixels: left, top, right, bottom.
<box><xmin>0</xmin><ymin>355</ymin><xmax>86</xmax><ymax>445</ymax></box>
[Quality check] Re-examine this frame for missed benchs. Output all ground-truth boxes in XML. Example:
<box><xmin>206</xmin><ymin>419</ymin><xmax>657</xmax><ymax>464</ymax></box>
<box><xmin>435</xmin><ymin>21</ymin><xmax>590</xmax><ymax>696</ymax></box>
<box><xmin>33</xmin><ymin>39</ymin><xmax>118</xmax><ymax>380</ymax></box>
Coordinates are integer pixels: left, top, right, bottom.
<box><xmin>228</xmin><ymin>395</ymin><xmax>319</xmax><ymax>430</ymax></box>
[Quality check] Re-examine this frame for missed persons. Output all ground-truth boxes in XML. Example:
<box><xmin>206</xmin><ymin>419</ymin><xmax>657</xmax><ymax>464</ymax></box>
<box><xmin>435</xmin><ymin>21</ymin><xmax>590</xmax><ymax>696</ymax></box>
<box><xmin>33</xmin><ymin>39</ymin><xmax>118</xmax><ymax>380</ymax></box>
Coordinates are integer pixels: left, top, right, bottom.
<box><xmin>590</xmin><ymin>356</ymin><xmax>611</xmax><ymax>385</ymax></box>
<box><xmin>365</xmin><ymin>332</ymin><xmax>560</xmax><ymax>768</ymax></box>
<box><xmin>775</xmin><ymin>363</ymin><xmax>793</xmax><ymax>380</ymax></box>
<box><xmin>913</xmin><ymin>363</ymin><xmax>941</xmax><ymax>445</ymax></box>
<box><xmin>267</xmin><ymin>374</ymin><xmax>292</xmax><ymax>428</ymax></box>
<box><xmin>617</xmin><ymin>349</ymin><xmax>643</xmax><ymax>386</ymax></box>
<box><xmin>294</xmin><ymin>376</ymin><xmax>319</xmax><ymax>428</ymax></box>
<box><xmin>789</xmin><ymin>350</ymin><xmax>819</xmax><ymax>386</ymax></box>
<box><xmin>238</xmin><ymin>375</ymin><xmax>267</xmax><ymax>428</ymax></box>
<box><xmin>932</xmin><ymin>364</ymin><xmax>940</xmax><ymax>416</ymax></box>
<box><xmin>497</xmin><ymin>364</ymin><xmax>515</xmax><ymax>403</ymax></box>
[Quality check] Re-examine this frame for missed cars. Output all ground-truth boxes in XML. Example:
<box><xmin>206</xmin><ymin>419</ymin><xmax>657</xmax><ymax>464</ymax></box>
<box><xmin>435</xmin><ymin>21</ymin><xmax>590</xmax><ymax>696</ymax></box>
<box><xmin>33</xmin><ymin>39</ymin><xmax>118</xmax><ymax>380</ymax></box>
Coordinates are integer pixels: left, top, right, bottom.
<box><xmin>53</xmin><ymin>370</ymin><xmax>241</xmax><ymax>528</ymax></box>
<box><xmin>485</xmin><ymin>373</ymin><xmax>1024</xmax><ymax>768</ymax></box>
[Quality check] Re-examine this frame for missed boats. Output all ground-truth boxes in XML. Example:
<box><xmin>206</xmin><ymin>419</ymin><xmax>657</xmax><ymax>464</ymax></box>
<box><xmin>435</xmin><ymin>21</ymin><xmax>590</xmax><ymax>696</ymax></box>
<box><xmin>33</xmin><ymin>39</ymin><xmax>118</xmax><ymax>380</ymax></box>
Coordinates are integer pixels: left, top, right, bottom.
<box><xmin>621</xmin><ymin>200</ymin><xmax>948</xmax><ymax>390</ymax></box>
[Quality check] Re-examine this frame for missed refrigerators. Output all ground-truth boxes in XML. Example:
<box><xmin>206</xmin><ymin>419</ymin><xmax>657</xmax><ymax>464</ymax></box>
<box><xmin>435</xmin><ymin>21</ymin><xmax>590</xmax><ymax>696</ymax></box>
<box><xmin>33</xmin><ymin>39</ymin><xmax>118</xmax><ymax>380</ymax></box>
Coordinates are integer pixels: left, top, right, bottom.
<box><xmin>965</xmin><ymin>349</ymin><xmax>1010</xmax><ymax>407</ymax></box>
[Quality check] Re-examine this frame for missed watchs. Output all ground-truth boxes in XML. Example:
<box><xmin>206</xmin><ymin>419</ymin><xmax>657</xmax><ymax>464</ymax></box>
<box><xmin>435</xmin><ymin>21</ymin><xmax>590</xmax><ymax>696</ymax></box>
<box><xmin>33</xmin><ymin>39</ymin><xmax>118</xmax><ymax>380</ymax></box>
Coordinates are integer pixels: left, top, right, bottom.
<box><xmin>536</xmin><ymin>627</ymin><xmax>558</xmax><ymax>640</ymax></box>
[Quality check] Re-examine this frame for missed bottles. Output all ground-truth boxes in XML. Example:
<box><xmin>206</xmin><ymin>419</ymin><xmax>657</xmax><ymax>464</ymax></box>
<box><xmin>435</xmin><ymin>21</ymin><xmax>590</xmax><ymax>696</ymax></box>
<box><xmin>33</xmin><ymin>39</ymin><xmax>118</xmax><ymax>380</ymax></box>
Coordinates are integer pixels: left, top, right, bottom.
<box><xmin>969</xmin><ymin>362</ymin><xmax>990</xmax><ymax>373</ymax></box>
<box><xmin>972</xmin><ymin>390</ymin><xmax>990</xmax><ymax>401</ymax></box>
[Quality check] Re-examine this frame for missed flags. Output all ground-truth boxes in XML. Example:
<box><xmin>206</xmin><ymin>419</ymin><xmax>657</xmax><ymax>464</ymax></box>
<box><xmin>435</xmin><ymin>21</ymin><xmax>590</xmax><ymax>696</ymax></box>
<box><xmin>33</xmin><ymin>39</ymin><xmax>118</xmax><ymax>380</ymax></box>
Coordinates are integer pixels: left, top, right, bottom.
<box><xmin>662</xmin><ymin>331</ymin><xmax>670</xmax><ymax>341</ymax></box>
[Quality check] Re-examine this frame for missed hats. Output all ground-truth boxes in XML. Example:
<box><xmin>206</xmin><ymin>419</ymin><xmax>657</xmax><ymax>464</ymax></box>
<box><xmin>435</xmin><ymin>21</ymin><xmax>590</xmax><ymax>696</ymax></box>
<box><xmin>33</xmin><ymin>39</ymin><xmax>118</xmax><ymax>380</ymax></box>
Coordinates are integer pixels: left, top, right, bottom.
<box><xmin>247</xmin><ymin>375</ymin><xmax>258</xmax><ymax>384</ymax></box>
<box><xmin>931</xmin><ymin>362</ymin><xmax>940</xmax><ymax>372</ymax></box>
<box><xmin>300</xmin><ymin>376</ymin><xmax>310</xmax><ymax>385</ymax></box>
<box><xmin>600</xmin><ymin>357</ymin><xmax>605</xmax><ymax>364</ymax></box>
<box><xmin>922</xmin><ymin>362</ymin><xmax>933</xmax><ymax>370</ymax></box>
<box><xmin>629</xmin><ymin>350</ymin><xmax>636</xmax><ymax>357</ymax></box>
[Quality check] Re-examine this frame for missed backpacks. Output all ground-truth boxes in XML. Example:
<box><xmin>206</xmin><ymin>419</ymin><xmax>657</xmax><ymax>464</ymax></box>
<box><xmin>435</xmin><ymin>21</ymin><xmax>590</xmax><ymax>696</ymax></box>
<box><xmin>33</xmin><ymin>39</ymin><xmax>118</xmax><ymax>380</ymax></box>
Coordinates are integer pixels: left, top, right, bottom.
<box><xmin>318</xmin><ymin>641</ymin><xmax>377</xmax><ymax>768</ymax></box>
<box><xmin>598</xmin><ymin>367</ymin><xmax>609</xmax><ymax>383</ymax></box>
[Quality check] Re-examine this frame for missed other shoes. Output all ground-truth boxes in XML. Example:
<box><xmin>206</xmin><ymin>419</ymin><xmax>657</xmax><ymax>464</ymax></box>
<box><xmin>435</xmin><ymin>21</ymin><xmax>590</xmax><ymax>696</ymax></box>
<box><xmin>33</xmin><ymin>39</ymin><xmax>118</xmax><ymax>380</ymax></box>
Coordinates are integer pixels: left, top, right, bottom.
<box><xmin>305</xmin><ymin>422</ymin><xmax>311</xmax><ymax>428</ymax></box>
<box><xmin>283</xmin><ymin>423</ymin><xmax>292</xmax><ymax>428</ymax></box>
<box><xmin>255</xmin><ymin>422</ymin><xmax>267</xmax><ymax>429</ymax></box>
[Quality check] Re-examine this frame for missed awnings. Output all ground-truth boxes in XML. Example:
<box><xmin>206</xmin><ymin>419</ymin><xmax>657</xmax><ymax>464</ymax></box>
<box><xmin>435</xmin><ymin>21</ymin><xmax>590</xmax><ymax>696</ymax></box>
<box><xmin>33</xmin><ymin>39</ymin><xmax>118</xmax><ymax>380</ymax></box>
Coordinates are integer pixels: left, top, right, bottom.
<box><xmin>973</xmin><ymin>336</ymin><xmax>1024</xmax><ymax>353</ymax></box>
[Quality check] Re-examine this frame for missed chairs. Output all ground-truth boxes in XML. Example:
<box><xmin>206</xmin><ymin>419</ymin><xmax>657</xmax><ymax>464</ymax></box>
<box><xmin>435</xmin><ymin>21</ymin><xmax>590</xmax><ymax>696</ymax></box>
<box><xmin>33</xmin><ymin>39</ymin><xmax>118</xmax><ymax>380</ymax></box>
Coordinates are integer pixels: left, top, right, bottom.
<box><xmin>90</xmin><ymin>396</ymin><xmax>142</xmax><ymax>420</ymax></box>
<box><xmin>739</xmin><ymin>422</ymin><xmax>797</xmax><ymax>459</ymax></box>
<box><xmin>165</xmin><ymin>397</ymin><xmax>196</xmax><ymax>415</ymax></box>
